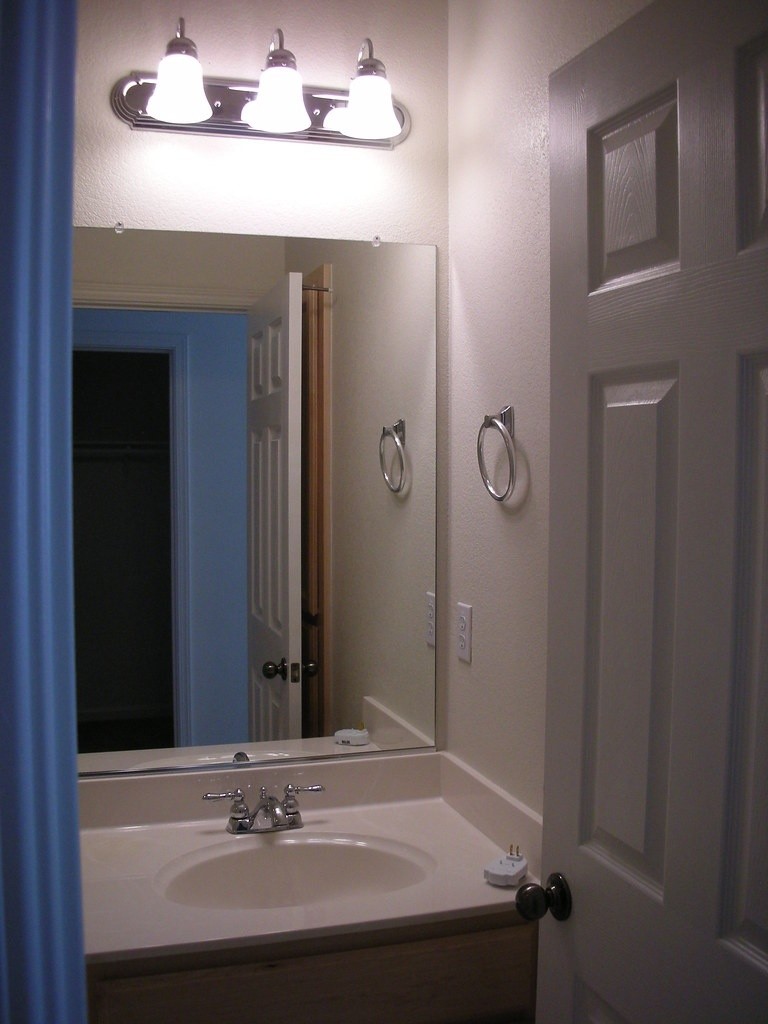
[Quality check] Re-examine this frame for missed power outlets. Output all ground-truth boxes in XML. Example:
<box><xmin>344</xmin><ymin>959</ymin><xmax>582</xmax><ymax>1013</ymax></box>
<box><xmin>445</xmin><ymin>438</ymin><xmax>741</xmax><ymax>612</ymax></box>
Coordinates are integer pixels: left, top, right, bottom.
<box><xmin>424</xmin><ymin>591</ymin><xmax>436</xmax><ymax>647</ymax></box>
<box><xmin>454</xmin><ymin>603</ymin><xmax>472</xmax><ymax>663</ymax></box>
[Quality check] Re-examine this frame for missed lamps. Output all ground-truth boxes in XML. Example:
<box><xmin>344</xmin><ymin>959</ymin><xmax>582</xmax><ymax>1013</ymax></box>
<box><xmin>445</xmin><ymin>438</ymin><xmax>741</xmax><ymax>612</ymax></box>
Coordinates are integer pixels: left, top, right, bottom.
<box><xmin>108</xmin><ymin>15</ymin><xmax>412</xmax><ymax>150</ymax></box>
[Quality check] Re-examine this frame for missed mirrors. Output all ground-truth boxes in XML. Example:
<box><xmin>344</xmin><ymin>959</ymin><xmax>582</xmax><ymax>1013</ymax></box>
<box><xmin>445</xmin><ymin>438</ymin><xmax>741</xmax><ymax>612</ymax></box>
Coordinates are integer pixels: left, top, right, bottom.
<box><xmin>73</xmin><ymin>225</ymin><xmax>439</xmax><ymax>780</ymax></box>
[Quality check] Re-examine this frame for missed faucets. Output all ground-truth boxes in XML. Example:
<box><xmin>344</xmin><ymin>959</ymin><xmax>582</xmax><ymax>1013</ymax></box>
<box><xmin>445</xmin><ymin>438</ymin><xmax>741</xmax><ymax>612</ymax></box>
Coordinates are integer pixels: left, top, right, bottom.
<box><xmin>249</xmin><ymin>794</ymin><xmax>288</xmax><ymax>831</ymax></box>
<box><xmin>233</xmin><ymin>750</ymin><xmax>249</xmax><ymax>763</ymax></box>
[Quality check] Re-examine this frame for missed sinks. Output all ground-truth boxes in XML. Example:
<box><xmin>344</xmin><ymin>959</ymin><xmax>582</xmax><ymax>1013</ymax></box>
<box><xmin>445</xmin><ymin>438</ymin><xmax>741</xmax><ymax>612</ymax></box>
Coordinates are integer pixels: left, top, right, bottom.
<box><xmin>149</xmin><ymin>821</ymin><xmax>444</xmax><ymax>919</ymax></box>
<box><xmin>130</xmin><ymin>747</ymin><xmax>317</xmax><ymax>772</ymax></box>
